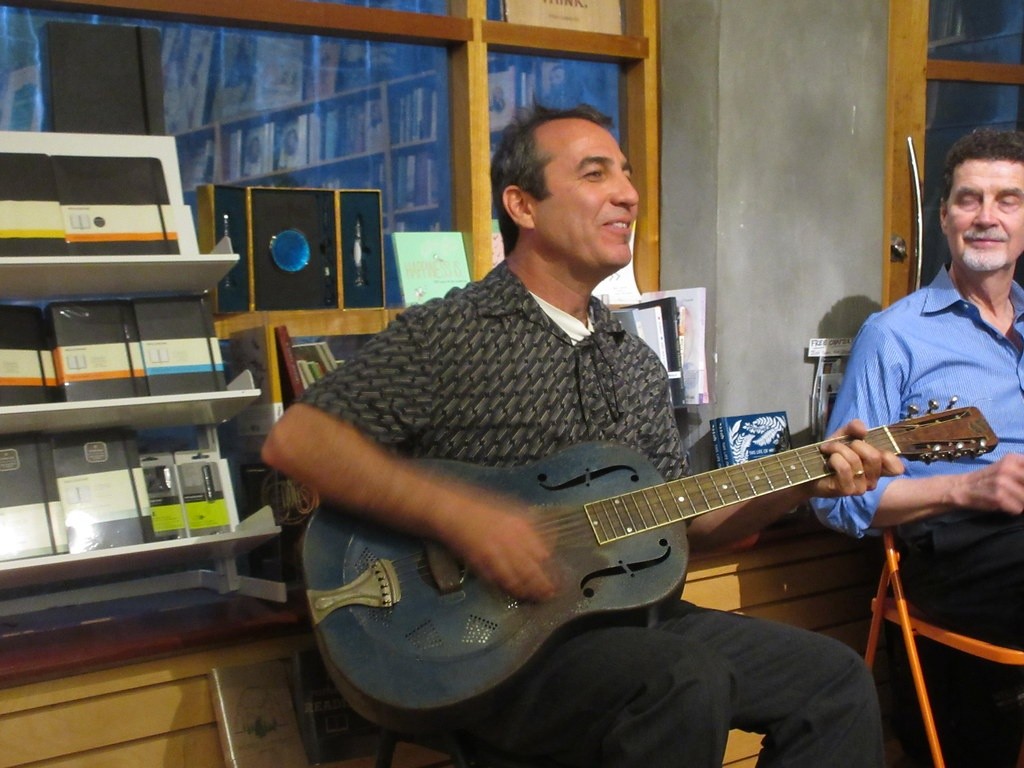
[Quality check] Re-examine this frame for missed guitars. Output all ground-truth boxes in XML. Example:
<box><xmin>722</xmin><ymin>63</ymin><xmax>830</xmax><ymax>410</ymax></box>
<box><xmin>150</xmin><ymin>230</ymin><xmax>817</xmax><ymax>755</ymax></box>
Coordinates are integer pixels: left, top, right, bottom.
<box><xmin>294</xmin><ymin>395</ymin><xmax>998</xmax><ymax>728</ymax></box>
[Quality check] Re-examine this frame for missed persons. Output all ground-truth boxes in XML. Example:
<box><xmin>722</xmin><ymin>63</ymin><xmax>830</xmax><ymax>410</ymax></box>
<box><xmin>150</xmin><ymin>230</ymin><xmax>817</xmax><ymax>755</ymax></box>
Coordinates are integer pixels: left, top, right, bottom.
<box><xmin>263</xmin><ymin>102</ymin><xmax>907</xmax><ymax>768</ymax></box>
<box><xmin>796</xmin><ymin>112</ymin><xmax>1021</xmax><ymax>544</ymax></box>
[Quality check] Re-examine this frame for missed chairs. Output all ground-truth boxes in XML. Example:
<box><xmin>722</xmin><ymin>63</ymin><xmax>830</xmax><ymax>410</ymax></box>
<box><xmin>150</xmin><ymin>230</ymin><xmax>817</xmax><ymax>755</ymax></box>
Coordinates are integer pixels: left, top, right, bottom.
<box><xmin>316</xmin><ymin>642</ymin><xmax>481</xmax><ymax>768</ymax></box>
<box><xmin>867</xmin><ymin>529</ymin><xmax>1024</xmax><ymax>768</ymax></box>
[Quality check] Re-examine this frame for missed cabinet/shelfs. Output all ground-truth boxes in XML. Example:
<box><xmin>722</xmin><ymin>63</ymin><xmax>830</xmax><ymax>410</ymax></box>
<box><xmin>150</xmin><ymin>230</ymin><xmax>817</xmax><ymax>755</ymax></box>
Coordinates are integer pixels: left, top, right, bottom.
<box><xmin>1</xmin><ymin>519</ymin><xmax>896</xmax><ymax>768</ymax></box>
<box><xmin>0</xmin><ymin>127</ymin><xmax>309</xmax><ymax>682</ymax></box>
<box><xmin>214</xmin><ymin>308</ymin><xmax>407</xmax><ymax>604</ymax></box>
<box><xmin>1</xmin><ymin>2</ymin><xmax>624</xmax><ymax>232</ymax></box>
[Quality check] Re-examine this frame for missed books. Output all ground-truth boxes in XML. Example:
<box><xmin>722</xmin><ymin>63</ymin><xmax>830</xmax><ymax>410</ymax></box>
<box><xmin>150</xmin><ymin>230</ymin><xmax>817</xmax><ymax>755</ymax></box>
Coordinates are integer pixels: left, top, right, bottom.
<box><xmin>168</xmin><ymin>15</ymin><xmax>588</xmax><ymax>207</ymax></box>
<box><xmin>0</xmin><ymin>420</ymin><xmax>157</xmax><ymax>564</ymax></box>
<box><xmin>229</xmin><ymin>321</ymin><xmax>387</xmax><ymax>514</ymax></box>
<box><xmin>1</xmin><ymin>298</ymin><xmax>227</xmax><ymax>406</ymax></box>
<box><xmin>608</xmin><ymin>285</ymin><xmax>710</xmax><ymax>408</ymax></box>
<box><xmin>702</xmin><ymin>410</ymin><xmax>793</xmax><ymax>468</ymax></box>
